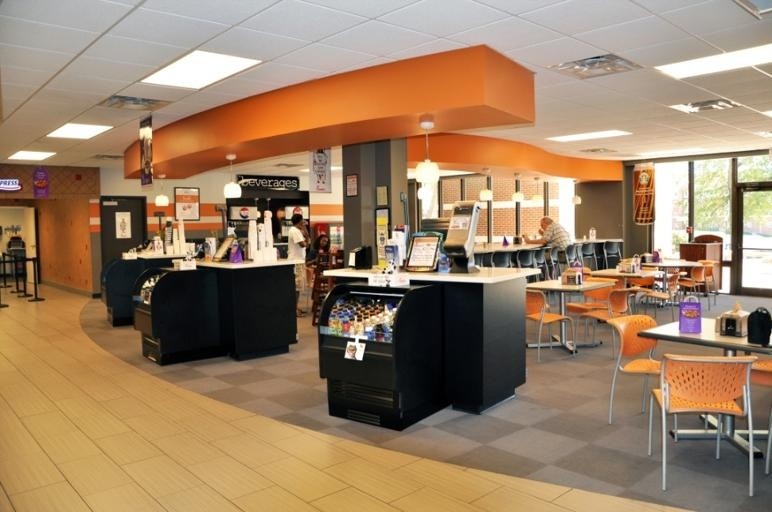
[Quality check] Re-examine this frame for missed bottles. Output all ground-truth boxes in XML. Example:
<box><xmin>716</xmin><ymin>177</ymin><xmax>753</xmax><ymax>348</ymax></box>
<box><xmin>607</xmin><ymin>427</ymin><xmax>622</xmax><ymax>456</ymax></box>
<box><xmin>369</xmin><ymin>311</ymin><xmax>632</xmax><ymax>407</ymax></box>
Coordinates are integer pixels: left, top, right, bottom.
<box><xmin>438</xmin><ymin>255</ymin><xmax>448</xmax><ymax>272</ymax></box>
<box><xmin>588</xmin><ymin>228</ymin><xmax>597</xmax><ymax>240</ymax></box>
<box><xmin>327</xmin><ymin>303</ymin><xmax>394</xmax><ymax>340</ymax></box>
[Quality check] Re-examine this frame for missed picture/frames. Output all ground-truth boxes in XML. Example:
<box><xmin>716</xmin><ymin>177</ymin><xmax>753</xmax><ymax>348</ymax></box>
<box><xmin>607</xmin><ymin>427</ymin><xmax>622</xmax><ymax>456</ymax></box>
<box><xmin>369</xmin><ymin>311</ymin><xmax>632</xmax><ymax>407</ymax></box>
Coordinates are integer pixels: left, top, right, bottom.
<box><xmin>346</xmin><ymin>174</ymin><xmax>358</xmax><ymax>197</ymax></box>
<box><xmin>376</xmin><ymin>185</ymin><xmax>389</xmax><ymax>206</ymax></box>
<box><xmin>173</xmin><ymin>186</ymin><xmax>200</xmax><ymax>222</ymax></box>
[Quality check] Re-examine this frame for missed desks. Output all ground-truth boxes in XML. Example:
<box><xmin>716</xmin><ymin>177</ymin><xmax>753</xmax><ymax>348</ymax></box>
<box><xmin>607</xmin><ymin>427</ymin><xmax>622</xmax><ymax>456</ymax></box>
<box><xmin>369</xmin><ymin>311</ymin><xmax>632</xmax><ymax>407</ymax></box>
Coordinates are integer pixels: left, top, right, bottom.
<box><xmin>136</xmin><ymin>253</ymin><xmax>187</xmax><ymax>269</ymax></box>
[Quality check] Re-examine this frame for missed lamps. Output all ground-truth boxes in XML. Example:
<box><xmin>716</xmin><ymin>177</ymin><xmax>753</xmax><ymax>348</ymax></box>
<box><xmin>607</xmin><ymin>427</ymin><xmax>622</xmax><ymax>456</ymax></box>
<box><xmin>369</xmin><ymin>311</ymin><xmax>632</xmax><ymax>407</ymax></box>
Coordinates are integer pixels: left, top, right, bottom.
<box><xmin>223</xmin><ymin>154</ymin><xmax>242</xmax><ymax>199</ymax></box>
<box><xmin>155</xmin><ymin>173</ymin><xmax>168</xmax><ymax>206</ymax></box>
<box><xmin>416</xmin><ymin>121</ymin><xmax>582</xmax><ymax>205</ymax></box>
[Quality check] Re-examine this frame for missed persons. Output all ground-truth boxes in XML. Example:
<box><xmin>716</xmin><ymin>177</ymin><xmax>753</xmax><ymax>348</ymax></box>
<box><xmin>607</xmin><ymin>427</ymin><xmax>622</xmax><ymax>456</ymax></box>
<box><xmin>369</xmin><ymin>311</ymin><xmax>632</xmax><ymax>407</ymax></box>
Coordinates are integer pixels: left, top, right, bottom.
<box><xmin>522</xmin><ymin>217</ymin><xmax>572</xmax><ymax>280</ymax></box>
<box><xmin>285</xmin><ymin>213</ymin><xmax>311</xmax><ymax>318</ymax></box>
<box><xmin>307</xmin><ymin>233</ymin><xmax>332</xmax><ymax>270</ymax></box>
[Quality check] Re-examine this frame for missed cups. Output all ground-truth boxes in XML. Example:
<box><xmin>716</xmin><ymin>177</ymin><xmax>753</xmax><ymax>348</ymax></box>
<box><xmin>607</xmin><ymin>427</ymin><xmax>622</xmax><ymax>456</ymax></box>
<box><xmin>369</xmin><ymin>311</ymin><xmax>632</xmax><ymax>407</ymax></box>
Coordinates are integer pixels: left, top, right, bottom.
<box><xmin>247</xmin><ymin>210</ymin><xmax>278</xmax><ymax>263</ymax></box>
<box><xmin>167</xmin><ymin>216</ymin><xmax>186</xmax><ymax>256</ymax></box>
